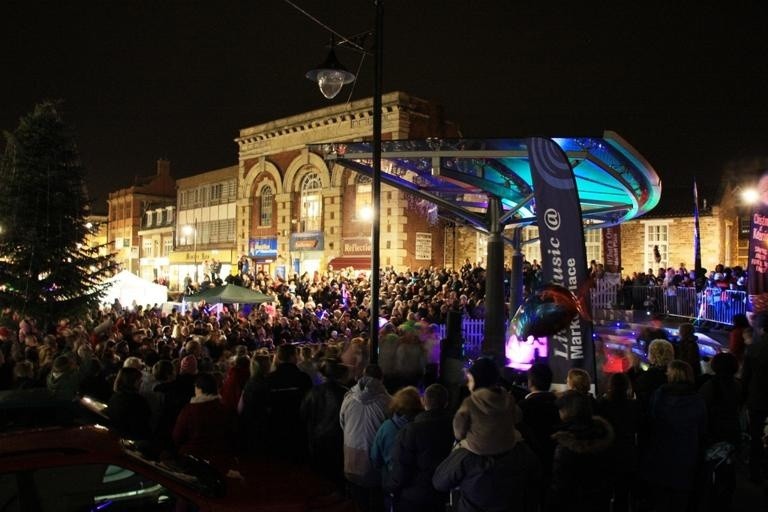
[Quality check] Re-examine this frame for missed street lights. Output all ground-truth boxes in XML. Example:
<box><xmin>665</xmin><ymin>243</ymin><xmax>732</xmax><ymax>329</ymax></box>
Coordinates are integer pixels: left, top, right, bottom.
<box><xmin>303</xmin><ymin>30</ymin><xmax>384</xmax><ymax>366</ymax></box>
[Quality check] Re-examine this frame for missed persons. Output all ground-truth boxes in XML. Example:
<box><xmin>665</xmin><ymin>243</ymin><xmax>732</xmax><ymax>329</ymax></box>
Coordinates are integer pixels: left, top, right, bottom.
<box><xmin>2</xmin><ymin>253</ymin><xmax>768</xmax><ymax>512</ymax></box>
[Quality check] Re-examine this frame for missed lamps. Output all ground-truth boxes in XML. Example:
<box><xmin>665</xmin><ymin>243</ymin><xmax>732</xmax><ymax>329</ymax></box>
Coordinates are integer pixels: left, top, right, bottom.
<box><xmin>304</xmin><ymin>32</ymin><xmax>356</xmax><ymax>101</ymax></box>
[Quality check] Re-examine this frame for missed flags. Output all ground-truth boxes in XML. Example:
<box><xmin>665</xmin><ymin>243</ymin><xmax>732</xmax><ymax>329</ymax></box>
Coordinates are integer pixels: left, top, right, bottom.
<box><xmin>692</xmin><ymin>200</ymin><xmax>704</xmax><ymax>279</ymax></box>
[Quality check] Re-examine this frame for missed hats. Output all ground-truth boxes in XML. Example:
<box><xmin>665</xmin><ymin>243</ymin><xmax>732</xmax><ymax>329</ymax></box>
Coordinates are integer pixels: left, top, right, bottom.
<box><xmin>180</xmin><ymin>354</ymin><xmax>197</xmax><ymax>373</ymax></box>
<box><xmin>469</xmin><ymin>357</ymin><xmax>499</xmax><ymax>391</ymax></box>
